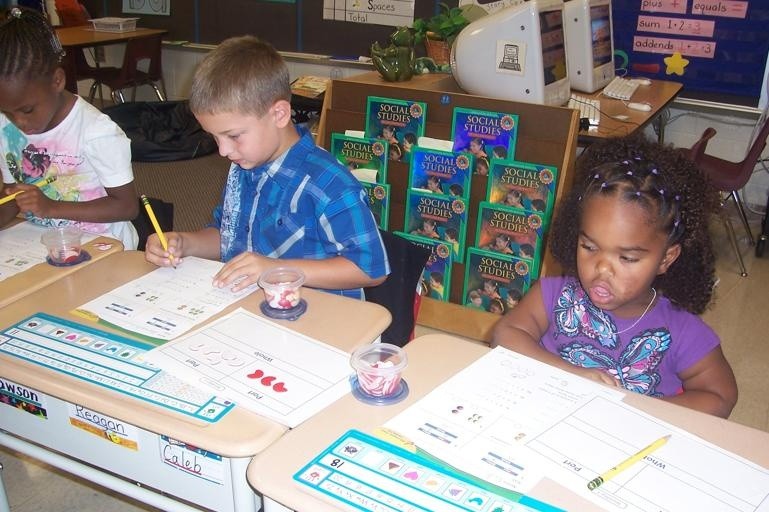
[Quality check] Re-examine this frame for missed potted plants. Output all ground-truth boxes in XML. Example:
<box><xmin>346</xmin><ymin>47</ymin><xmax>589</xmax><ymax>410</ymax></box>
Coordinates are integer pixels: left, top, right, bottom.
<box><xmin>413</xmin><ymin>2</ymin><xmax>466</xmax><ymax>72</ymax></box>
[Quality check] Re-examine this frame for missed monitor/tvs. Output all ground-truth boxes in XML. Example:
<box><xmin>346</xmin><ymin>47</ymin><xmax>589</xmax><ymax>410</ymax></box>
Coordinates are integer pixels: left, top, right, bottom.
<box><xmin>564</xmin><ymin>1</ymin><xmax>615</xmax><ymax>96</ymax></box>
<box><xmin>448</xmin><ymin>1</ymin><xmax>570</xmax><ymax>109</ymax></box>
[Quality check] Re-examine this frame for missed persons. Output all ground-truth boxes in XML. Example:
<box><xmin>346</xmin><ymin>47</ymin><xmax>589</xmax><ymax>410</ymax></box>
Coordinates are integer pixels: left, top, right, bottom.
<box><xmin>144</xmin><ymin>32</ymin><xmax>395</xmax><ymax>303</ymax></box>
<box><xmin>0</xmin><ymin>6</ymin><xmax>142</xmax><ymax>255</ymax></box>
<box><xmin>481</xmin><ymin>133</ymin><xmax>737</xmax><ymax>419</ymax></box>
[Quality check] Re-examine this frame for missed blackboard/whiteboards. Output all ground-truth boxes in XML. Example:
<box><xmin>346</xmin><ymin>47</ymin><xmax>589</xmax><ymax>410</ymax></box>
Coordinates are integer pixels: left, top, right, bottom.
<box><xmin>93</xmin><ymin>0</ymin><xmax>497</xmax><ymax>65</ymax></box>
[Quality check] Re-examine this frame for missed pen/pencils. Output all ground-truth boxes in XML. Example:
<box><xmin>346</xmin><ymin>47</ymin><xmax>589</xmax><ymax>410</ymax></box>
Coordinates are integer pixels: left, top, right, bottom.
<box><xmin>0</xmin><ymin>175</ymin><xmax>58</xmax><ymax>205</ymax></box>
<box><xmin>588</xmin><ymin>434</ymin><xmax>672</xmax><ymax>490</ymax></box>
<box><xmin>140</xmin><ymin>194</ymin><xmax>177</xmax><ymax>269</ymax></box>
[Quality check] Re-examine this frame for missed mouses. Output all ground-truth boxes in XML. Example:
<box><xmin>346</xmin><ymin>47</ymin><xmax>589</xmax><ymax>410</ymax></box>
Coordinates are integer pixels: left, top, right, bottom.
<box><xmin>628</xmin><ymin>103</ymin><xmax>653</xmax><ymax>112</ymax></box>
<box><xmin>631</xmin><ymin>78</ymin><xmax>653</xmax><ymax>86</ymax></box>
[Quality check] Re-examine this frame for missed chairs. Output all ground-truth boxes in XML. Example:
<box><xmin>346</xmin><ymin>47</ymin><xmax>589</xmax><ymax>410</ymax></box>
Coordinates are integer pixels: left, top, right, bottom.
<box><xmin>78</xmin><ymin>31</ymin><xmax>167</xmax><ymax>117</ymax></box>
<box><xmin>670</xmin><ymin>113</ymin><xmax>769</xmax><ymax>277</ymax></box>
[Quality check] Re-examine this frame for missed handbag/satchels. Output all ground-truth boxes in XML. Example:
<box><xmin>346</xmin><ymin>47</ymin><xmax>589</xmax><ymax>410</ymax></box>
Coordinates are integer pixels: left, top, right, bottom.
<box><xmin>100</xmin><ymin>99</ymin><xmax>218</xmax><ymax>163</ymax></box>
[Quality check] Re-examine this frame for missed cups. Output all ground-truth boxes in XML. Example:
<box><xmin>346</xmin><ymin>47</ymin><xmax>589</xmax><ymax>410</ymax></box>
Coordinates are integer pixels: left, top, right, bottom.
<box><xmin>349</xmin><ymin>341</ymin><xmax>409</xmax><ymax>393</ymax></box>
<box><xmin>257</xmin><ymin>266</ymin><xmax>305</xmax><ymax>308</ymax></box>
<box><xmin>40</xmin><ymin>226</ymin><xmax>84</xmax><ymax>262</ymax></box>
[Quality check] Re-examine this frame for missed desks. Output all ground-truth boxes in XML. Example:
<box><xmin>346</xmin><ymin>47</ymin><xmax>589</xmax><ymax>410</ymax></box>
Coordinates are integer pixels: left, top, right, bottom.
<box><xmin>562</xmin><ymin>72</ymin><xmax>685</xmax><ymax>156</ymax></box>
<box><xmin>48</xmin><ymin>22</ymin><xmax>169</xmax><ymax>113</ymax></box>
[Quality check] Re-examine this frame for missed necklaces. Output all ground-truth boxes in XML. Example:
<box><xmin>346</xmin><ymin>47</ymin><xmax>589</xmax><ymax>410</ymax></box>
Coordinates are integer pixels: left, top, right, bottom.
<box><xmin>578</xmin><ymin>289</ymin><xmax>658</xmax><ymax>339</ymax></box>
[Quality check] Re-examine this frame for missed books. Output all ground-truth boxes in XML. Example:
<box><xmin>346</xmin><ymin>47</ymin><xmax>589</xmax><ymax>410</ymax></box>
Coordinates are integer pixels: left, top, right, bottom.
<box><xmin>331</xmin><ymin>90</ymin><xmax>560</xmax><ymax>317</ymax></box>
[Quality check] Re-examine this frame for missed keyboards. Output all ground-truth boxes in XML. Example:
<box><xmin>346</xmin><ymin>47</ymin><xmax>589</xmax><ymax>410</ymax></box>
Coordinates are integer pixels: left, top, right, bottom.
<box><xmin>602</xmin><ymin>76</ymin><xmax>639</xmax><ymax>101</ymax></box>
<box><xmin>567</xmin><ymin>94</ymin><xmax>602</xmax><ymax>127</ymax></box>
